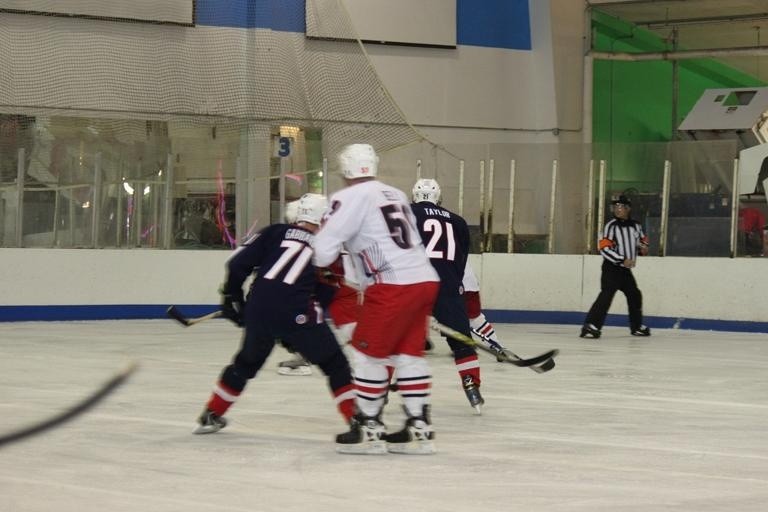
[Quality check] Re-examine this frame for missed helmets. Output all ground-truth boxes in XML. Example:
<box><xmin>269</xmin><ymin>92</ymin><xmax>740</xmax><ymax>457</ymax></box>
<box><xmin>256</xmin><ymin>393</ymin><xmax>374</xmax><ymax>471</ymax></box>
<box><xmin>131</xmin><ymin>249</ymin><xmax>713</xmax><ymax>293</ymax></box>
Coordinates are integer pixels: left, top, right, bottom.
<box><xmin>296</xmin><ymin>193</ymin><xmax>328</xmax><ymax>226</ymax></box>
<box><xmin>337</xmin><ymin>143</ymin><xmax>379</xmax><ymax>180</ymax></box>
<box><xmin>285</xmin><ymin>200</ymin><xmax>298</xmax><ymax>224</ymax></box>
<box><xmin>412</xmin><ymin>178</ymin><xmax>443</xmax><ymax>206</ymax></box>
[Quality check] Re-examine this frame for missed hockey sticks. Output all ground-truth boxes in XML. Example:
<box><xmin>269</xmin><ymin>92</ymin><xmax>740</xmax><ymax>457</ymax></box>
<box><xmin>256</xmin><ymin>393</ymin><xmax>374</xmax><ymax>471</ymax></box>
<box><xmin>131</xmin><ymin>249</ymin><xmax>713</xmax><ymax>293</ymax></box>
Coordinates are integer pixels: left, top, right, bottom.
<box><xmin>166</xmin><ymin>304</ymin><xmax>222</xmax><ymax>327</ymax></box>
<box><xmin>430</xmin><ymin>317</ymin><xmax>560</xmax><ymax>373</ymax></box>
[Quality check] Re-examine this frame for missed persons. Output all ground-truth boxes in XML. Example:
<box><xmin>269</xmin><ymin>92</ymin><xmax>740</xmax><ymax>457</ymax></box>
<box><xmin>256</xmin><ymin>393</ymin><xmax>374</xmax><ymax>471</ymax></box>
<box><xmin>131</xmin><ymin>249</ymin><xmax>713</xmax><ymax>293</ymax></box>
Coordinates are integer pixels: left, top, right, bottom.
<box><xmin>736</xmin><ymin>207</ymin><xmax>767</xmax><ymax>257</ymax></box>
<box><xmin>584</xmin><ymin>197</ymin><xmax>649</xmax><ymax>333</ymax></box>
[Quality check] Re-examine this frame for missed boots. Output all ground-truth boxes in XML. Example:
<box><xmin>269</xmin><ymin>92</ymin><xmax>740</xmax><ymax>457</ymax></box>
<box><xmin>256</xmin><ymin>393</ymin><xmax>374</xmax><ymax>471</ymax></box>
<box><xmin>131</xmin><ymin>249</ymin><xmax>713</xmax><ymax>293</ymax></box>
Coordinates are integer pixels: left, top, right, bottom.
<box><xmin>279</xmin><ymin>353</ymin><xmax>309</xmax><ymax>367</ymax></box>
<box><xmin>632</xmin><ymin>324</ymin><xmax>649</xmax><ymax>335</ymax></box>
<box><xmin>492</xmin><ymin>343</ymin><xmax>507</xmax><ymax>363</ymax></box>
<box><xmin>196</xmin><ymin>409</ymin><xmax>227</xmax><ymax>427</ymax></box>
<box><xmin>387</xmin><ymin>405</ymin><xmax>434</xmax><ymax>442</ymax></box>
<box><xmin>462</xmin><ymin>375</ymin><xmax>484</xmax><ymax>406</ymax></box>
<box><xmin>336</xmin><ymin>412</ymin><xmax>387</xmax><ymax>444</ymax></box>
<box><xmin>583</xmin><ymin>322</ymin><xmax>600</xmax><ymax>336</ymax></box>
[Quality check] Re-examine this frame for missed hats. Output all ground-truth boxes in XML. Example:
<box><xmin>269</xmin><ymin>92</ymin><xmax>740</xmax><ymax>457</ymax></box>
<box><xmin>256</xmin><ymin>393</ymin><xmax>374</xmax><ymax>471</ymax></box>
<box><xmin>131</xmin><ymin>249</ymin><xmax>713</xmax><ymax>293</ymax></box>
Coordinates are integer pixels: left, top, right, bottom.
<box><xmin>612</xmin><ymin>195</ymin><xmax>633</xmax><ymax>207</ymax></box>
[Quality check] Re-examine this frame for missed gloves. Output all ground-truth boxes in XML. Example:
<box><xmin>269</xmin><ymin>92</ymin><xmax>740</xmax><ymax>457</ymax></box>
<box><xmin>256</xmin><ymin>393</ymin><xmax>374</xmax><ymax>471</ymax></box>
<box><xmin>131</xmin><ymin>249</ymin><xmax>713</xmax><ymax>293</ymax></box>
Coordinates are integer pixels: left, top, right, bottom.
<box><xmin>221</xmin><ymin>290</ymin><xmax>246</xmax><ymax>327</ymax></box>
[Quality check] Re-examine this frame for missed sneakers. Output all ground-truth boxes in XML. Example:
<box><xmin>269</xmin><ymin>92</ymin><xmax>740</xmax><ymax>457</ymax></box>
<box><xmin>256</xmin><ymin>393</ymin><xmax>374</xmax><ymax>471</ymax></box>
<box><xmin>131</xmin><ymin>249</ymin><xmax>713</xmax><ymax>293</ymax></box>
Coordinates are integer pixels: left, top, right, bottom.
<box><xmin>349</xmin><ymin>413</ymin><xmax>364</xmax><ymax>430</ymax></box>
